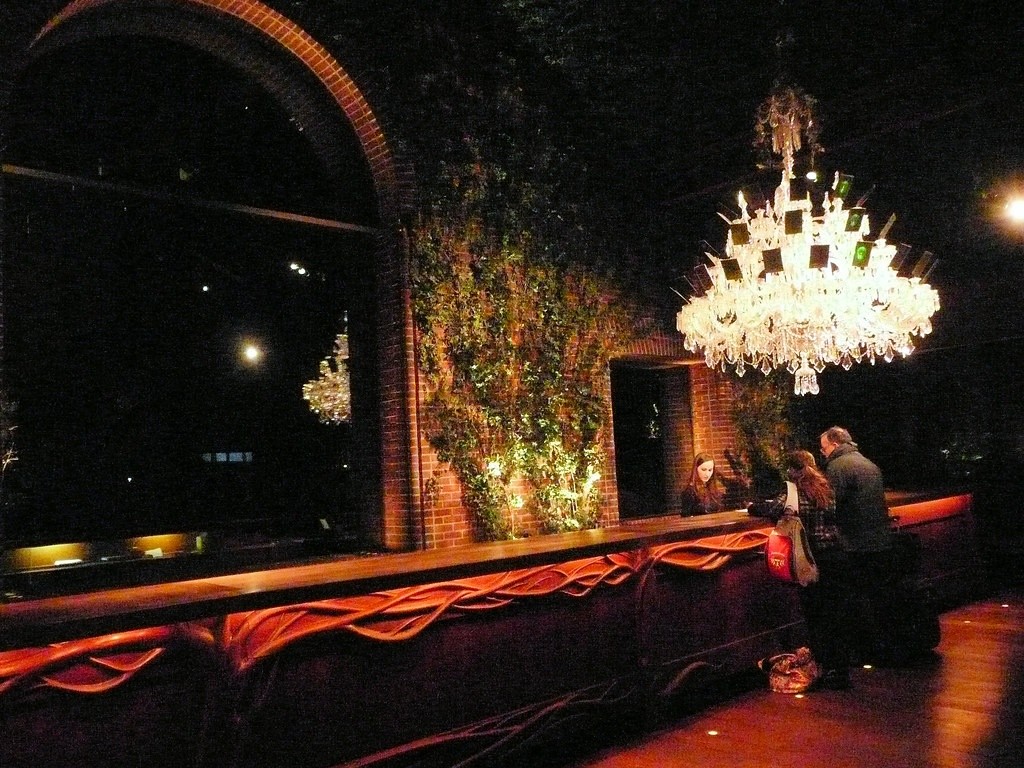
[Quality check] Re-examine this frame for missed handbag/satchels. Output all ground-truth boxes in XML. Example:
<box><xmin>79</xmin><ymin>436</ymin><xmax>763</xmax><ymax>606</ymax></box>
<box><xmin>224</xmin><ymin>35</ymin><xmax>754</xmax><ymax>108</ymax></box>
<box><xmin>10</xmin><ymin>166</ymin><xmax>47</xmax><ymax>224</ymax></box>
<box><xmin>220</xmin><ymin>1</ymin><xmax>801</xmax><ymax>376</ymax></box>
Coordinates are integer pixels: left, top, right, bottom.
<box><xmin>768</xmin><ymin>481</ymin><xmax>819</xmax><ymax>587</ymax></box>
<box><xmin>769</xmin><ymin>647</ymin><xmax>823</xmax><ymax>694</ymax></box>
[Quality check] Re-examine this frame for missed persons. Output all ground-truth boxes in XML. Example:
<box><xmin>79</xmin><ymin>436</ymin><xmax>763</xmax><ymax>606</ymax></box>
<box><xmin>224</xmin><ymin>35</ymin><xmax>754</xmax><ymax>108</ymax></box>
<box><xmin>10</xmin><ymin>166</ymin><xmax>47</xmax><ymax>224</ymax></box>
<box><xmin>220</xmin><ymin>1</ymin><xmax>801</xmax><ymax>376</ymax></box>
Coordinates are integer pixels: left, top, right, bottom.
<box><xmin>745</xmin><ymin>450</ymin><xmax>852</xmax><ymax>689</ymax></box>
<box><xmin>681</xmin><ymin>452</ymin><xmax>722</xmax><ymax>518</ymax></box>
<box><xmin>819</xmin><ymin>425</ymin><xmax>900</xmax><ymax>665</ymax></box>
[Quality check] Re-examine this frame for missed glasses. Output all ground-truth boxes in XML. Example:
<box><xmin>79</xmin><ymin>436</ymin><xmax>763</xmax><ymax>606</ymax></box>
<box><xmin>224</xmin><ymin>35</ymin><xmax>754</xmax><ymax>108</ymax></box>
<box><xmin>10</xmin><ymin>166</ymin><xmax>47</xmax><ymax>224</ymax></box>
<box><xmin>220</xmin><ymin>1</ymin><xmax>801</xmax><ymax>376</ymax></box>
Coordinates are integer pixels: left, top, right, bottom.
<box><xmin>820</xmin><ymin>440</ymin><xmax>835</xmax><ymax>454</ymax></box>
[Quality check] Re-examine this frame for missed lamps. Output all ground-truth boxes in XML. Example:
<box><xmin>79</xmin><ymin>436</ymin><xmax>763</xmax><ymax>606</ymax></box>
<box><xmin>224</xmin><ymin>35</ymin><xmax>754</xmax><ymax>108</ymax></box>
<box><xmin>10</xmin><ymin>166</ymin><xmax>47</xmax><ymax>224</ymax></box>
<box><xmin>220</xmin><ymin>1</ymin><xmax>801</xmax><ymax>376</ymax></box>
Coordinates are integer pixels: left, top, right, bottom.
<box><xmin>302</xmin><ymin>309</ymin><xmax>350</xmax><ymax>428</ymax></box>
<box><xmin>669</xmin><ymin>25</ymin><xmax>941</xmax><ymax>394</ymax></box>
